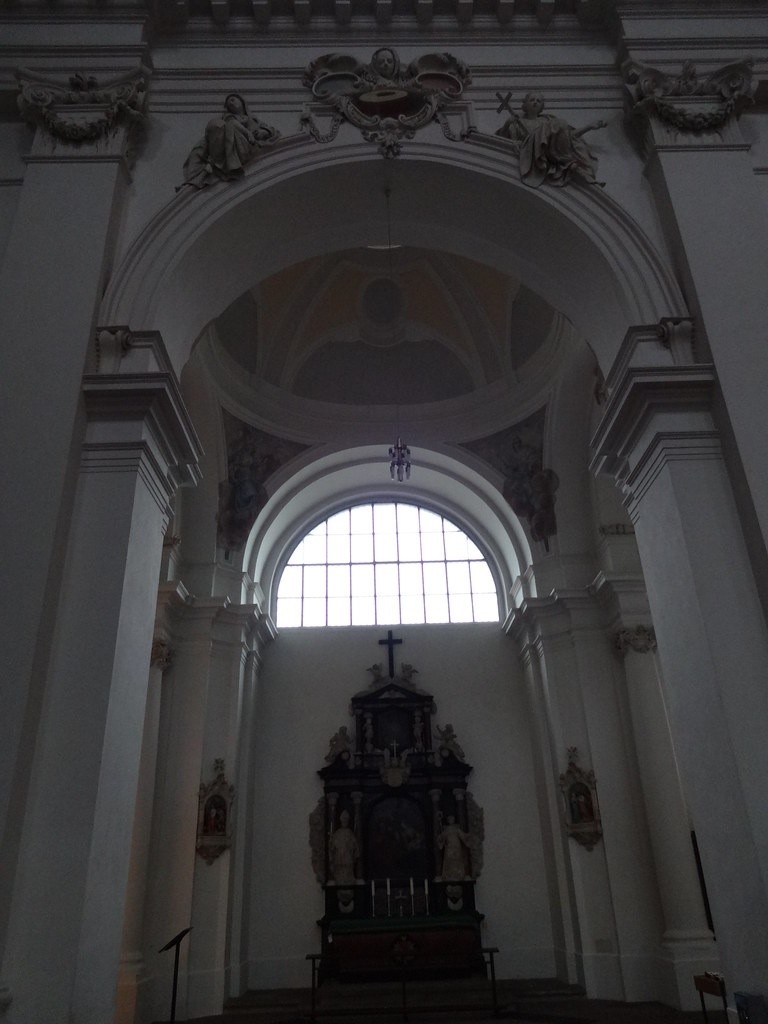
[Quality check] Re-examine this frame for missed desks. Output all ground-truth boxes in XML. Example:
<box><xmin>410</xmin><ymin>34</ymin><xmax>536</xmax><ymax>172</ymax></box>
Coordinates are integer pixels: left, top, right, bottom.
<box><xmin>306</xmin><ymin>947</ymin><xmax>500</xmax><ymax>1014</ymax></box>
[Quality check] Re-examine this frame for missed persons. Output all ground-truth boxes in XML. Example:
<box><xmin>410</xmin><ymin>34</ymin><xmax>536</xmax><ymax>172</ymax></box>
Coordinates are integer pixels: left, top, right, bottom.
<box><xmin>205</xmin><ymin>91</ymin><xmax>276</xmax><ymax>181</ymax></box>
<box><xmin>495</xmin><ymin>89</ymin><xmax>607</xmax><ymax>188</ymax></box>
<box><xmin>324</xmin><ymin>725</ymin><xmax>354</xmax><ymax>768</ymax></box>
<box><xmin>370</xmin><ymin>45</ymin><xmax>399</xmax><ymax>77</ymax></box>
<box><xmin>433</xmin><ymin>723</ymin><xmax>466</xmax><ymax>765</ymax></box>
<box><xmin>435</xmin><ymin>815</ymin><xmax>466</xmax><ymax>882</ymax></box>
<box><xmin>327</xmin><ymin>809</ymin><xmax>361</xmax><ymax>887</ymax></box>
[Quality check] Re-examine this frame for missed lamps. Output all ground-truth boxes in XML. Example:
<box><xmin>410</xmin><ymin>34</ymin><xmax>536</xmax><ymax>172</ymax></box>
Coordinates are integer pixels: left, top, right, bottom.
<box><xmin>389</xmin><ymin>437</ymin><xmax>411</xmax><ymax>482</ymax></box>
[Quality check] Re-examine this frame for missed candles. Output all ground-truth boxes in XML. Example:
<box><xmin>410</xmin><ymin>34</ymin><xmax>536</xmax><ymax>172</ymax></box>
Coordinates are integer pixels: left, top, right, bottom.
<box><xmin>410</xmin><ymin>877</ymin><xmax>414</xmax><ymax>895</ymax></box>
<box><xmin>387</xmin><ymin>878</ymin><xmax>390</xmax><ymax>895</ymax></box>
<box><xmin>372</xmin><ymin>880</ymin><xmax>375</xmax><ymax>896</ymax></box>
<box><xmin>425</xmin><ymin>879</ymin><xmax>428</xmax><ymax>894</ymax></box>
